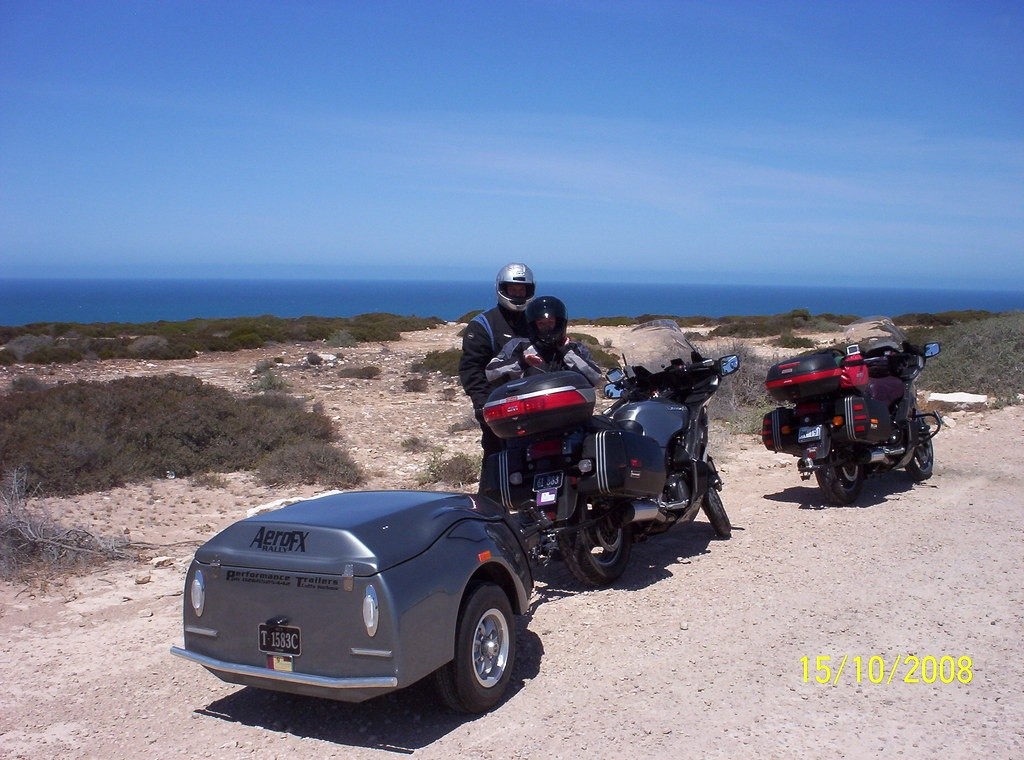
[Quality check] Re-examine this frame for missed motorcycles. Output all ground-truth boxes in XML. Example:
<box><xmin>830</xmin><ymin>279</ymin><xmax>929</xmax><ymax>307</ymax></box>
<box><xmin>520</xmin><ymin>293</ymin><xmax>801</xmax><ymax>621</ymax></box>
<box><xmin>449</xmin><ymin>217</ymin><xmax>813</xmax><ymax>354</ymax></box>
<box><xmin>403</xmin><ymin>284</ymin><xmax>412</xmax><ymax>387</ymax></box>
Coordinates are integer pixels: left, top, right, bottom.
<box><xmin>479</xmin><ymin>319</ymin><xmax>738</xmax><ymax>588</ymax></box>
<box><xmin>761</xmin><ymin>315</ymin><xmax>942</xmax><ymax>505</ymax></box>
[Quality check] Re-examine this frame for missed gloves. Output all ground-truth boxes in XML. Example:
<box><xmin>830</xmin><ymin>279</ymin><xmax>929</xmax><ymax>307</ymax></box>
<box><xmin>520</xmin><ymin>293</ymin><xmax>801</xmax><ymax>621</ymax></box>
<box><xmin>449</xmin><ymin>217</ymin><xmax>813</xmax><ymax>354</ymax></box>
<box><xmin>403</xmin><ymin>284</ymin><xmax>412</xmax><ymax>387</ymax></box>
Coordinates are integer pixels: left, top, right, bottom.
<box><xmin>475</xmin><ymin>408</ymin><xmax>487</xmax><ymax>425</ymax></box>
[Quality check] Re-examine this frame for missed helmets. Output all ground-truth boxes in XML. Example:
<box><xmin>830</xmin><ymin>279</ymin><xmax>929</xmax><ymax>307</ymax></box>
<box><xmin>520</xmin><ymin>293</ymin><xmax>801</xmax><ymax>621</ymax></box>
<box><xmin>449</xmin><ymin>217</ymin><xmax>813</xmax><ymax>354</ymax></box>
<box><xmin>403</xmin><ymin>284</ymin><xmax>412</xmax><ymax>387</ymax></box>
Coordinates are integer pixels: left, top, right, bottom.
<box><xmin>525</xmin><ymin>295</ymin><xmax>568</xmax><ymax>349</ymax></box>
<box><xmin>495</xmin><ymin>262</ymin><xmax>538</xmax><ymax>311</ymax></box>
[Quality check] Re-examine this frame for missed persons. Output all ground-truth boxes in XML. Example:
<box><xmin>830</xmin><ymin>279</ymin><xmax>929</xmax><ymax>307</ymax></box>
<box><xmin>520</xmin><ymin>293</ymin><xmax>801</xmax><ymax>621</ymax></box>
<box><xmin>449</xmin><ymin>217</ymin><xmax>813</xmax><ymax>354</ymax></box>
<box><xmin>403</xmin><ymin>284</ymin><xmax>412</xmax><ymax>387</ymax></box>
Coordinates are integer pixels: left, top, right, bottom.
<box><xmin>484</xmin><ymin>296</ymin><xmax>605</xmax><ymax>391</ymax></box>
<box><xmin>459</xmin><ymin>263</ymin><xmax>535</xmax><ymax>512</ymax></box>
